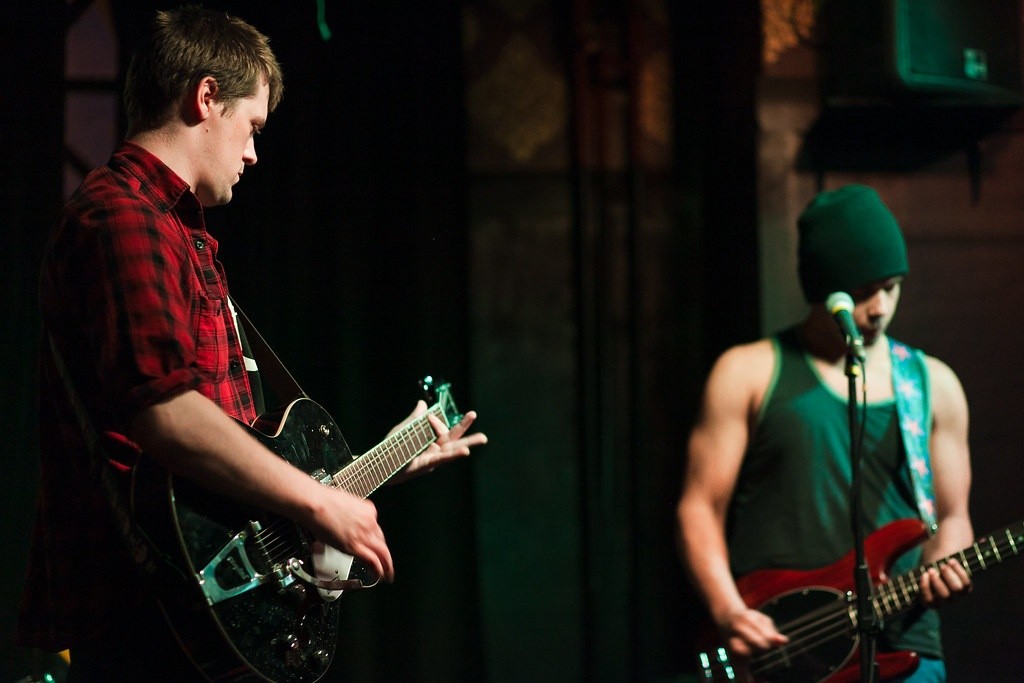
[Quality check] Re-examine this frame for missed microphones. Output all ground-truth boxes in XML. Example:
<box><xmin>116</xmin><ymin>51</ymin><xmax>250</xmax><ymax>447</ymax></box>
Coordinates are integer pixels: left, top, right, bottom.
<box><xmin>825</xmin><ymin>290</ymin><xmax>866</xmax><ymax>362</ymax></box>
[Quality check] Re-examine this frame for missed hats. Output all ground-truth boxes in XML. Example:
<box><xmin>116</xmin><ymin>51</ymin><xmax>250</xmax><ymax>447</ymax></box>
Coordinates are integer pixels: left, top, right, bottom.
<box><xmin>794</xmin><ymin>184</ymin><xmax>910</xmax><ymax>300</ymax></box>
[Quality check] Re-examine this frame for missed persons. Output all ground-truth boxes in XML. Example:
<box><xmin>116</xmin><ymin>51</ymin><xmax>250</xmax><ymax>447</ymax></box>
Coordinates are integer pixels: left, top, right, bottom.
<box><xmin>13</xmin><ymin>5</ymin><xmax>487</xmax><ymax>682</ymax></box>
<box><xmin>676</xmin><ymin>185</ymin><xmax>974</xmax><ymax>683</ymax></box>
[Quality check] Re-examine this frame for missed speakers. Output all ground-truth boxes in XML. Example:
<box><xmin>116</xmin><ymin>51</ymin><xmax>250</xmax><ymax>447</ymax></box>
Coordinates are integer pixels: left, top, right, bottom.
<box><xmin>816</xmin><ymin>0</ymin><xmax>1024</xmax><ymax>114</ymax></box>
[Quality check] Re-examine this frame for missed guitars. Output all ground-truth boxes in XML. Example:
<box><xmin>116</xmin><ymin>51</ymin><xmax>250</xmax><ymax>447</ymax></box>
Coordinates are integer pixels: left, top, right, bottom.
<box><xmin>138</xmin><ymin>375</ymin><xmax>466</xmax><ymax>683</ymax></box>
<box><xmin>685</xmin><ymin>516</ymin><xmax>1024</xmax><ymax>683</ymax></box>
